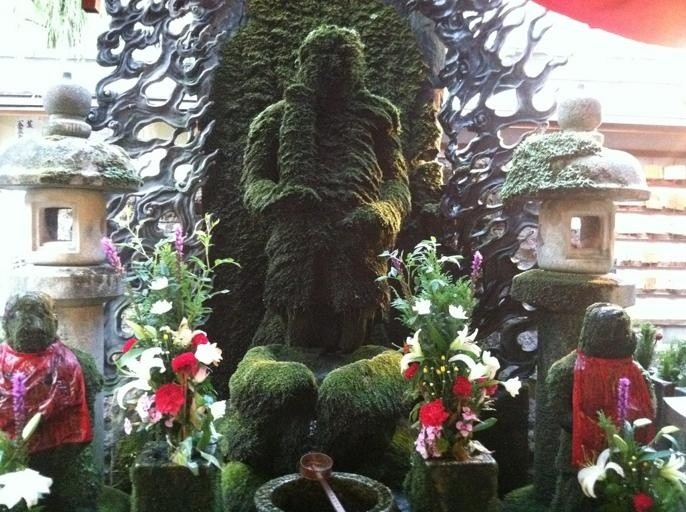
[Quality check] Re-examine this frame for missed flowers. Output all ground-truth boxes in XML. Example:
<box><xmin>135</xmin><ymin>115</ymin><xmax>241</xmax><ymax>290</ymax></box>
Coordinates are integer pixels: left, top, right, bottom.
<box><xmin>576</xmin><ymin>377</ymin><xmax>686</xmax><ymax>511</ymax></box>
<box><xmin>100</xmin><ymin>213</ymin><xmax>242</xmax><ymax>479</ymax></box>
<box><xmin>374</xmin><ymin>236</ymin><xmax>521</xmax><ymax>462</ymax></box>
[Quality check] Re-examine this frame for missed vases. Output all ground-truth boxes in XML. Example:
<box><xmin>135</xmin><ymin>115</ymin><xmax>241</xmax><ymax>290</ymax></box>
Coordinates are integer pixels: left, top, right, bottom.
<box><xmin>131</xmin><ymin>453</ymin><xmax>222</xmax><ymax>511</ymax></box>
<box><xmin>409</xmin><ymin>440</ymin><xmax>500</xmax><ymax>511</ymax></box>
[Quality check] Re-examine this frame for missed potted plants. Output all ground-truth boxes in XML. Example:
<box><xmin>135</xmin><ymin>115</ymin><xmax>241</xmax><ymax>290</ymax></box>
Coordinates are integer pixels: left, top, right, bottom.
<box><xmin>634</xmin><ymin>321</ymin><xmax>686</xmax><ymax>395</ymax></box>
